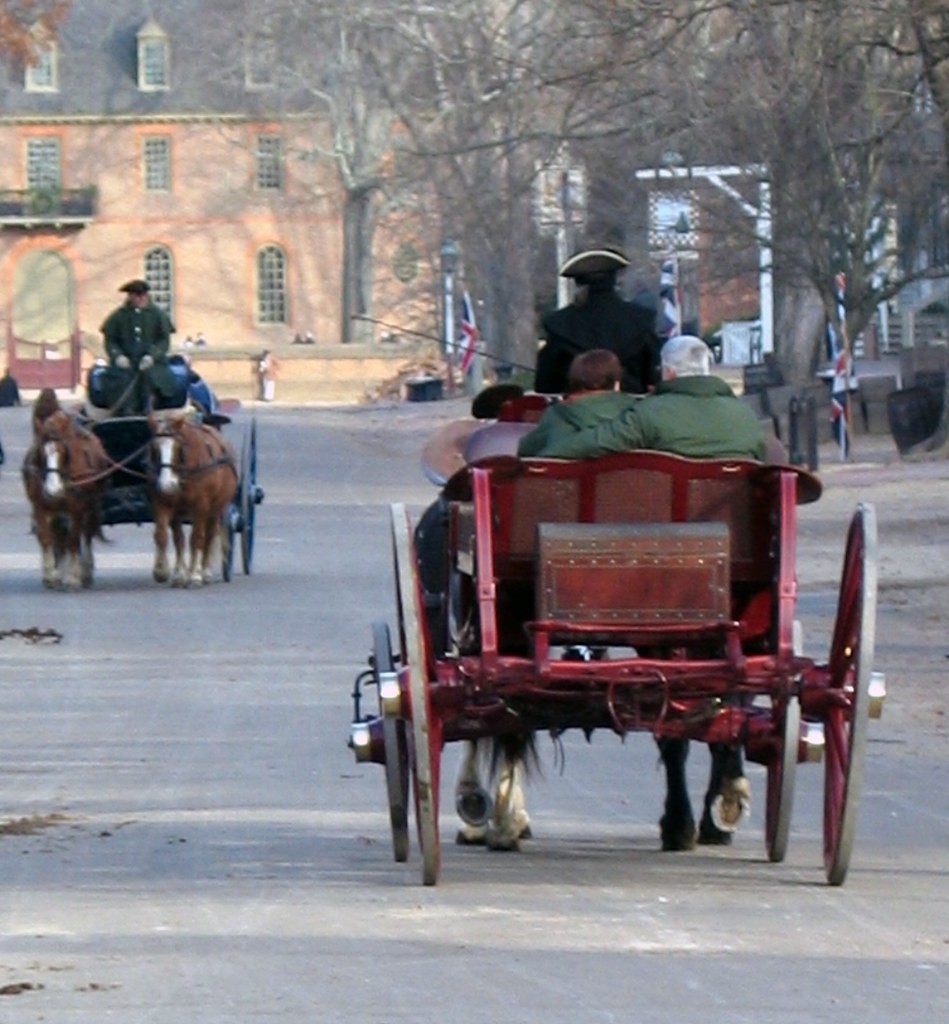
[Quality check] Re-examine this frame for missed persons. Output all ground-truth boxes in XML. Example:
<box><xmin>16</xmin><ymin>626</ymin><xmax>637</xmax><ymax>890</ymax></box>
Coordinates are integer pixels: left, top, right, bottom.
<box><xmin>537</xmin><ymin>336</ymin><xmax>767</xmax><ymax>459</ymax></box>
<box><xmin>184</xmin><ymin>332</ymin><xmax>205</xmax><ymax>348</ymax></box>
<box><xmin>100</xmin><ymin>279</ymin><xmax>177</xmax><ymax>416</ymax></box>
<box><xmin>291</xmin><ymin>332</ymin><xmax>313</xmax><ymax>343</ymax></box>
<box><xmin>258</xmin><ymin>350</ymin><xmax>275</xmax><ymax>401</ymax></box>
<box><xmin>518</xmin><ymin>350</ymin><xmax>639</xmax><ymax>458</ymax></box>
<box><xmin>534</xmin><ymin>248</ymin><xmax>669</xmax><ymax>395</ymax></box>
<box><xmin>0</xmin><ymin>367</ymin><xmax>21</xmax><ymax>407</ymax></box>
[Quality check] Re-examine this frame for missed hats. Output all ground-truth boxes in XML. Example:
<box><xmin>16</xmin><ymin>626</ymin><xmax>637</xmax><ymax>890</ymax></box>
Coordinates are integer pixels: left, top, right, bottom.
<box><xmin>558</xmin><ymin>240</ymin><xmax>632</xmax><ymax>277</ymax></box>
<box><xmin>118</xmin><ymin>279</ymin><xmax>150</xmax><ymax>292</ymax></box>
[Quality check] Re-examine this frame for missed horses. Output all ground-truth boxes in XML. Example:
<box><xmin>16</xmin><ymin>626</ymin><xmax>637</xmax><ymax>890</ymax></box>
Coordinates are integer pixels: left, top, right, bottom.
<box><xmin>147</xmin><ymin>395</ymin><xmax>239</xmax><ymax>590</ymax></box>
<box><xmin>24</xmin><ymin>417</ymin><xmax>108</xmax><ymax>589</ymax></box>
<box><xmin>453</xmin><ymin>421</ymin><xmax>791</xmax><ymax>853</ymax></box>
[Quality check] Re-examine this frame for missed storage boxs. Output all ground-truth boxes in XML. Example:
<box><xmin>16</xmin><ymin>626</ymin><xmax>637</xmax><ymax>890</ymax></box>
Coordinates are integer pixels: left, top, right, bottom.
<box><xmin>536</xmin><ymin>523</ymin><xmax>730</xmax><ymax>642</ymax></box>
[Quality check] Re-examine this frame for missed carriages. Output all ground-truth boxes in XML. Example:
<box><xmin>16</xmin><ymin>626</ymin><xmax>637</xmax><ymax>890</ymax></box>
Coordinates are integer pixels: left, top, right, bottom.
<box><xmin>19</xmin><ymin>354</ymin><xmax>266</xmax><ymax>590</ymax></box>
<box><xmin>348</xmin><ymin>358</ymin><xmax>887</xmax><ymax>886</ymax></box>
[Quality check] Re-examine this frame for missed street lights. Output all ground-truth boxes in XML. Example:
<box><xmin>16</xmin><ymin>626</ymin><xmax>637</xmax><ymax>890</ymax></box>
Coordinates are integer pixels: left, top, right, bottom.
<box><xmin>438</xmin><ymin>239</ymin><xmax>459</xmax><ymax>398</ymax></box>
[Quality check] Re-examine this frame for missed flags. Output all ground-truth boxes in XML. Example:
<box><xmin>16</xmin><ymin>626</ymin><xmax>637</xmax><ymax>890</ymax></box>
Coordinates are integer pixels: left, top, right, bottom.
<box><xmin>830</xmin><ymin>329</ymin><xmax>859</xmax><ymax>462</ymax></box>
<box><xmin>454</xmin><ymin>293</ymin><xmax>480</xmax><ymax>372</ymax></box>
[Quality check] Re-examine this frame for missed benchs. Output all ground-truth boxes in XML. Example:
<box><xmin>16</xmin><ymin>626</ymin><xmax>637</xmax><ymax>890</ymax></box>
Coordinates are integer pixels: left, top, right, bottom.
<box><xmin>413</xmin><ymin>449</ymin><xmax>823</xmax><ymax>591</ymax></box>
<box><xmin>85</xmin><ymin>353</ymin><xmax>191</xmax><ymax>419</ymax></box>
